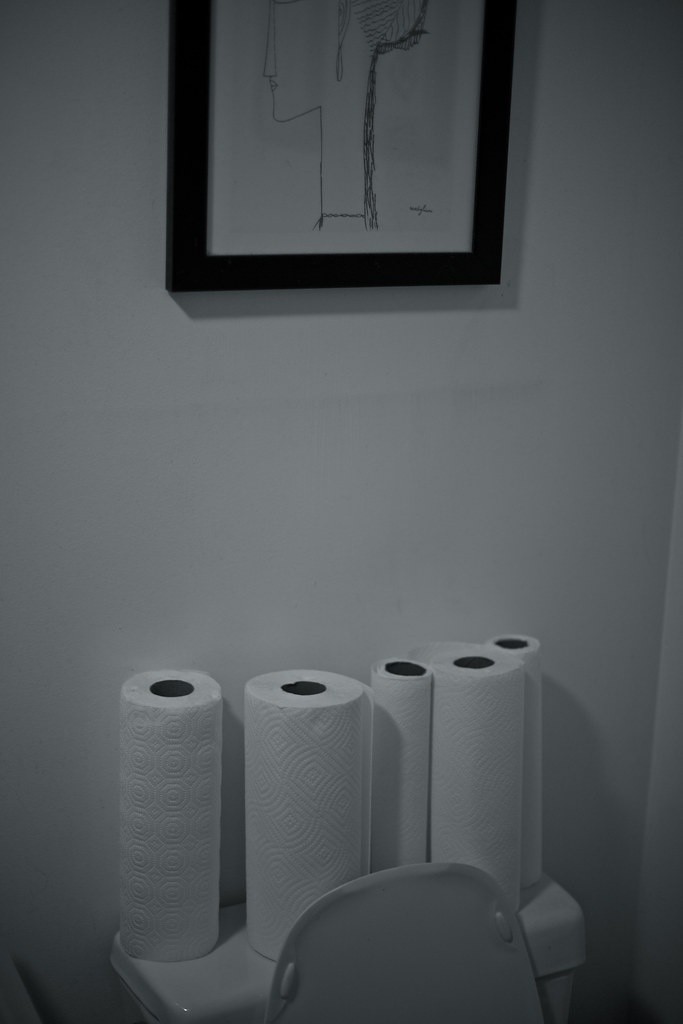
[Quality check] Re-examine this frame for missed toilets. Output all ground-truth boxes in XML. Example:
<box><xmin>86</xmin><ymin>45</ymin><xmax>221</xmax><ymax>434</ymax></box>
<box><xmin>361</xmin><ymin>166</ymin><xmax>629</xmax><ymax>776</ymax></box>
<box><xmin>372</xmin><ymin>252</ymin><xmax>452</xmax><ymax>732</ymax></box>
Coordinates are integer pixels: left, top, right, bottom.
<box><xmin>107</xmin><ymin>861</ymin><xmax>588</xmax><ymax>1024</ymax></box>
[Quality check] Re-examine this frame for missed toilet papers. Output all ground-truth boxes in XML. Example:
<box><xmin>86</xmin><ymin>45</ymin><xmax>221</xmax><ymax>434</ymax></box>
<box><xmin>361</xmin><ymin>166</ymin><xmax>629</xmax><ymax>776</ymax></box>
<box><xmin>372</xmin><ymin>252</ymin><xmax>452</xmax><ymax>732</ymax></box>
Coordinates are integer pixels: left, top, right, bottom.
<box><xmin>119</xmin><ymin>634</ymin><xmax>542</xmax><ymax>962</ymax></box>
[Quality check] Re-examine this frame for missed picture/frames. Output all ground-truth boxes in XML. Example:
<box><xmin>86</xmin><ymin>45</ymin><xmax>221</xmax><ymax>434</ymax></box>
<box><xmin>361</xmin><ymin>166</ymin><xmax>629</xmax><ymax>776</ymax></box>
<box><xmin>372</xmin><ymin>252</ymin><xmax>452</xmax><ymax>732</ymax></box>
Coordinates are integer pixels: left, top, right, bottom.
<box><xmin>164</xmin><ymin>0</ymin><xmax>517</xmax><ymax>293</ymax></box>
